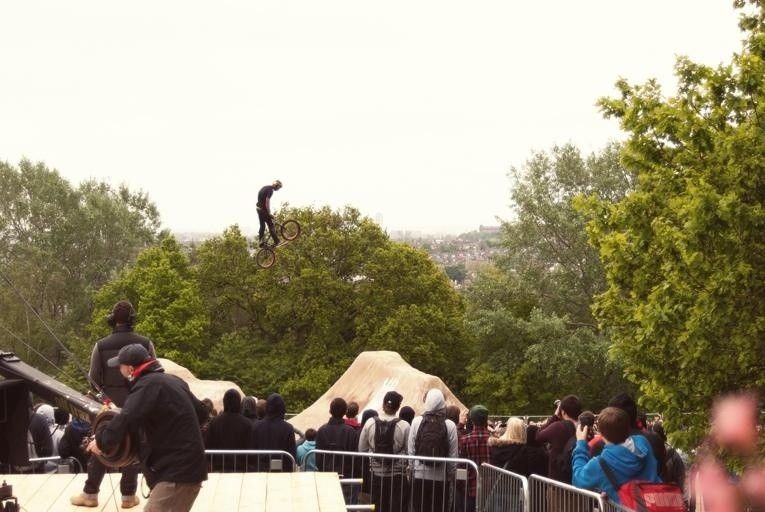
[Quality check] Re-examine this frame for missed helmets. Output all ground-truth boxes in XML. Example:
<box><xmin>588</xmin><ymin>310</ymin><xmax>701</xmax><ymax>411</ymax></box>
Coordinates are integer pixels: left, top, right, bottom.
<box><xmin>273</xmin><ymin>180</ymin><xmax>283</xmax><ymax>187</ymax></box>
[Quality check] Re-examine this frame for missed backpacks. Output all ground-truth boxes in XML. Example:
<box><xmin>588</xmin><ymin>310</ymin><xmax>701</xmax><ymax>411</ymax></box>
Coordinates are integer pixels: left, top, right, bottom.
<box><xmin>374</xmin><ymin>419</ymin><xmax>397</xmax><ymax>463</ymax></box>
<box><xmin>621</xmin><ymin>480</ymin><xmax>687</xmax><ymax>512</ymax></box>
<box><xmin>415</xmin><ymin>413</ymin><xmax>451</xmax><ymax>465</ymax></box>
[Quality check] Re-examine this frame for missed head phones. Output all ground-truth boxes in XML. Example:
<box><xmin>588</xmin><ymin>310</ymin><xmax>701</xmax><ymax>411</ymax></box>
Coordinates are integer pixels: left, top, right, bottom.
<box><xmin>107</xmin><ymin>303</ymin><xmax>136</xmax><ymax>327</ymax></box>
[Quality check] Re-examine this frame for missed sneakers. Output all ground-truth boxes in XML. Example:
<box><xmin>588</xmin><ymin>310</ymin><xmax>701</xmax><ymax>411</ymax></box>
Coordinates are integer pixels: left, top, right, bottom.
<box><xmin>120</xmin><ymin>494</ymin><xmax>141</xmax><ymax>508</ymax></box>
<box><xmin>70</xmin><ymin>493</ymin><xmax>100</xmax><ymax>507</ymax></box>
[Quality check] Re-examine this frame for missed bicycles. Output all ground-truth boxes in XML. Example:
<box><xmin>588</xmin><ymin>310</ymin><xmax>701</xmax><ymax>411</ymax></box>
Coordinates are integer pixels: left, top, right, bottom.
<box><xmin>255</xmin><ymin>214</ymin><xmax>302</xmax><ymax>270</ymax></box>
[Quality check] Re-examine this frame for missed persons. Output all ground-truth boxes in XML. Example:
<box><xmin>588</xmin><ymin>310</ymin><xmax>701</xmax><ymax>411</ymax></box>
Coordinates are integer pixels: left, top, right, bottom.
<box><xmin>255</xmin><ymin>178</ymin><xmax>283</xmax><ymax>250</ymax></box>
<box><xmin>69</xmin><ymin>299</ymin><xmax>158</xmax><ymax>509</ymax></box>
<box><xmin>83</xmin><ymin>342</ymin><xmax>209</xmax><ymax>512</ymax></box>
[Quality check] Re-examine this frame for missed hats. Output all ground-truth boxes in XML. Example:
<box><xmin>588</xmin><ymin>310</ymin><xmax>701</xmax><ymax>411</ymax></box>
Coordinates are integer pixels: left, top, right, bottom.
<box><xmin>471</xmin><ymin>404</ymin><xmax>489</xmax><ymax>425</ymax></box>
<box><xmin>384</xmin><ymin>391</ymin><xmax>403</xmax><ymax>410</ymax></box>
<box><xmin>106</xmin><ymin>342</ymin><xmax>153</xmax><ymax>367</ymax></box>
